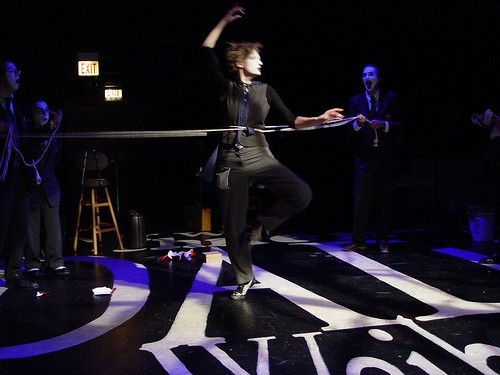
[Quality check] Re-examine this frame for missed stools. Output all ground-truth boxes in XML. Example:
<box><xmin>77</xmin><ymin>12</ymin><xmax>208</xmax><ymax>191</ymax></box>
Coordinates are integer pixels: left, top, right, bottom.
<box><xmin>72</xmin><ymin>153</ymin><xmax>123</xmax><ymax>257</ymax></box>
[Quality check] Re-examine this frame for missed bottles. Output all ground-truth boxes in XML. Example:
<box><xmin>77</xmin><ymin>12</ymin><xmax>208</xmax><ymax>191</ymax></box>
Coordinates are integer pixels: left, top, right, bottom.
<box><xmin>131</xmin><ymin>213</ymin><xmax>146</xmax><ymax>249</ymax></box>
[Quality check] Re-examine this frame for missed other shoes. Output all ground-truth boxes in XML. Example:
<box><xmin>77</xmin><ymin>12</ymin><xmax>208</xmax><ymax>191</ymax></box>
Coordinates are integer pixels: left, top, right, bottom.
<box><xmin>24</xmin><ymin>268</ymin><xmax>42</xmax><ymax>277</ymax></box>
<box><xmin>56</xmin><ymin>266</ymin><xmax>71</xmax><ymax>274</ymax></box>
<box><xmin>378</xmin><ymin>237</ymin><xmax>389</xmax><ymax>252</ymax></box>
<box><xmin>345</xmin><ymin>241</ymin><xmax>367</xmax><ymax>250</ymax></box>
<box><xmin>6</xmin><ymin>274</ymin><xmax>40</xmax><ymax>290</ymax></box>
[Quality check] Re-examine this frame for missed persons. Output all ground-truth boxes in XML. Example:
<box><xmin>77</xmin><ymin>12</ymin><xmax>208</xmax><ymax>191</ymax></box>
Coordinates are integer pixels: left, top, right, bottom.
<box><xmin>342</xmin><ymin>63</ymin><xmax>402</xmax><ymax>253</ymax></box>
<box><xmin>0</xmin><ymin>60</ymin><xmax>72</xmax><ymax>290</ymax></box>
<box><xmin>194</xmin><ymin>5</ymin><xmax>346</xmax><ymax>301</ymax></box>
<box><xmin>480</xmin><ymin>109</ymin><xmax>500</xmax><ymax>263</ymax></box>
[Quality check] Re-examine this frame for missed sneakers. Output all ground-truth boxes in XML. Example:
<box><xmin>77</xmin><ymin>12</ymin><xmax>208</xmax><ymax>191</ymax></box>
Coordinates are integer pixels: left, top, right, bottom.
<box><xmin>231</xmin><ymin>277</ymin><xmax>255</xmax><ymax>300</ymax></box>
